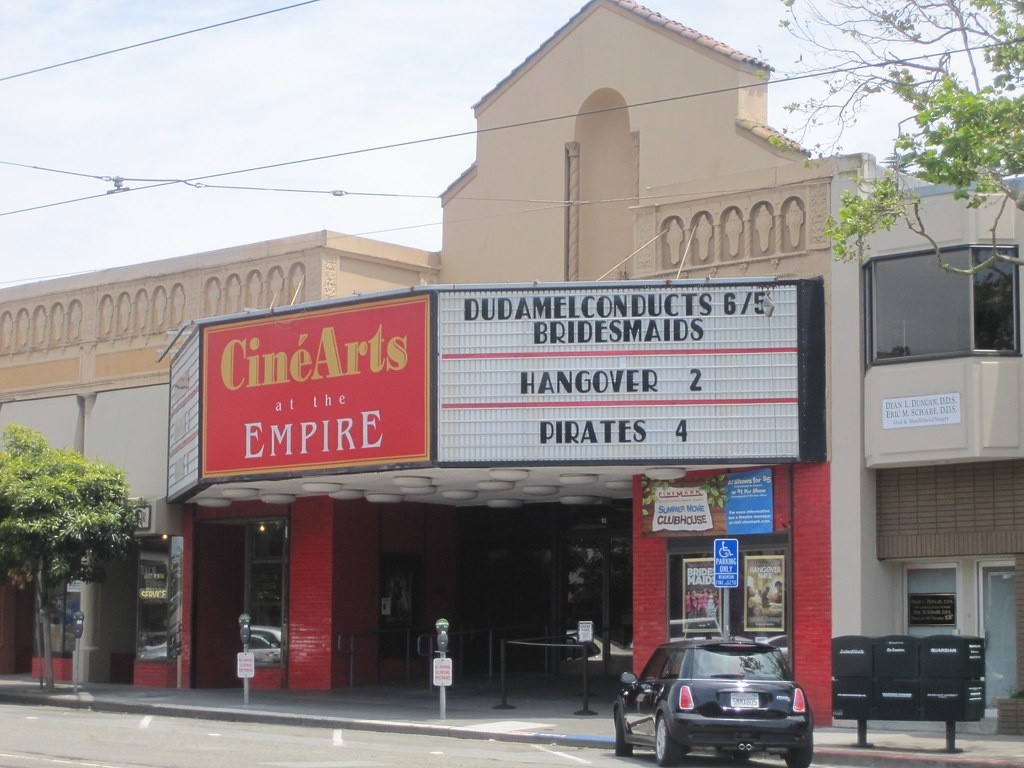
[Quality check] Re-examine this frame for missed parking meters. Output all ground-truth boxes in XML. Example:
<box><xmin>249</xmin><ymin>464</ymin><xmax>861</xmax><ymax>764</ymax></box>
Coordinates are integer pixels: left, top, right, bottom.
<box><xmin>239</xmin><ymin>612</ymin><xmax>251</xmax><ymax>704</ymax></box>
<box><xmin>436</xmin><ymin>619</ymin><xmax>449</xmax><ymax>718</ymax></box>
<box><xmin>72</xmin><ymin>611</ymin><xmax>84</xmax><ymax>695</ymax></box>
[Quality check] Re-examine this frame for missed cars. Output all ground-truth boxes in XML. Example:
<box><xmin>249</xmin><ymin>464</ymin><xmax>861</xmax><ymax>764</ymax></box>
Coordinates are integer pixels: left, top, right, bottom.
<box><xmin>612</xmin><ymin>640</ymin><xmax>813</xmax><ymax>768</ymax></box>
<box><xmin>566</xmin><ymin>630</ymin><xmax>633</xmax><ymax>660</ymax></box>
<box><xmin>138</xmin><ymin>632</ymin><xmax>167</xmax><ymax>659</ymax></box>
<box><xmin>250</xmin><ymin>625</ymin><xmax>281</xmax><ymax>664</ymax></box>
